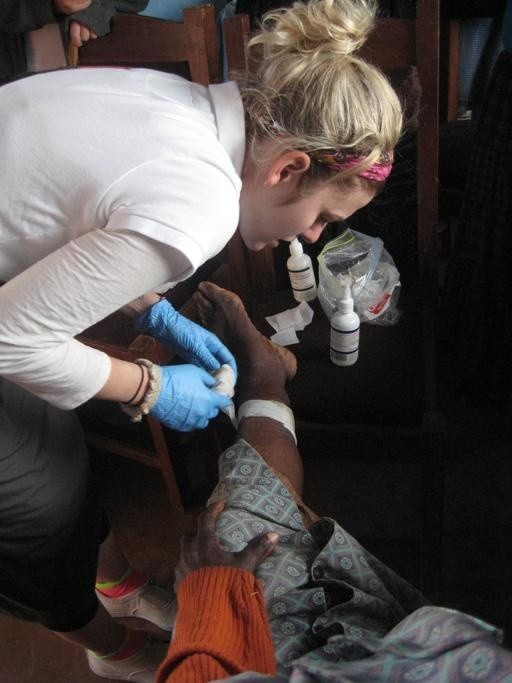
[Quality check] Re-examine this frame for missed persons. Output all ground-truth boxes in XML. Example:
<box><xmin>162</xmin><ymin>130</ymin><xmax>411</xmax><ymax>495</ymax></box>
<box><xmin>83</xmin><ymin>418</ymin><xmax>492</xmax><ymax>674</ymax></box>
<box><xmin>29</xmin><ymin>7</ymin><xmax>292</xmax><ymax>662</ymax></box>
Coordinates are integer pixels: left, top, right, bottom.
<box><xmin>2</xmin><ymin>52</ymin><xmax>403</xmax><ymax>680</ymax></box>
<box><xmin>3</xmin><ymin>0</ymin><xmax>150</xmax><ymax>49</ymax></box>
<box><xmin>157</xmin><ymin>279</ymin><xmax>509</xmax><ymax>679</ymax></box>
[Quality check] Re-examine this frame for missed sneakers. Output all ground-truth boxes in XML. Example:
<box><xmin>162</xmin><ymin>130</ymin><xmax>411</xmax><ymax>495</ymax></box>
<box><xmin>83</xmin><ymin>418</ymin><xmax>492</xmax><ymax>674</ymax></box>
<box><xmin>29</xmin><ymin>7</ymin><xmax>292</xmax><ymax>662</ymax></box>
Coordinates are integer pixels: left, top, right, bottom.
<box><xmin>88</xmin><ymin>631</ymin><xmax>170</xmax><ymax>681</ymax></box>
<box><xmin>94</xmin><ymin>574</ymin><xmax>180</xmax><ymax>631</ymax></box>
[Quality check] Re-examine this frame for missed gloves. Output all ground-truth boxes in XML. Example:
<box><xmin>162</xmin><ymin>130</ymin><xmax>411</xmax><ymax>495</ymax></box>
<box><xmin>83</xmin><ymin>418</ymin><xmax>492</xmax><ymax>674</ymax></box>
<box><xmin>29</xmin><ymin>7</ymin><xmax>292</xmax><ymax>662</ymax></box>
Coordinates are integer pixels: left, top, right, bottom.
<box><xmin>146</xmin><ymin>363</ymin><xmax>233</xmax><ymax>434</ymax></box>
<box><xmin>133</xmin><ymin>299</ymin><xmax>238</xmax><ymax>389</ymax></box>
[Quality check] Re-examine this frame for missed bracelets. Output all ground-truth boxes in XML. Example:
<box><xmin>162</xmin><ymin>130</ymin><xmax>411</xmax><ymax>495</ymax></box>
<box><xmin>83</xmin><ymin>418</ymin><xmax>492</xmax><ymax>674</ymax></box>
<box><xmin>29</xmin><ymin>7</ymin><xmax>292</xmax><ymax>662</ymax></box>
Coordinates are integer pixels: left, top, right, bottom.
<box><xmin>122</xmin><ymin>362</ymin><xmax>144</xmax><ymax>404</ymax></box>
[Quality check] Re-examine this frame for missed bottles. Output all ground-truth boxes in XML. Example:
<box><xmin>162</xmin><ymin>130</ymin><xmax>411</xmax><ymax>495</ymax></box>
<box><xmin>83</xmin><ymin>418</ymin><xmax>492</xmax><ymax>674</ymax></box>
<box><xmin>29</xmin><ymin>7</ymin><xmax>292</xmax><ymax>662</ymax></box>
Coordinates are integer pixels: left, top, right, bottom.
<box><xmin>287</xmin><ymin>237</ymin><xmax>317</xmax><ymax>302</ymax></box>
<box><xmin>330</xmin><ymin>286</ymin><xmax>362</xmax><ymax>367</ymax></box>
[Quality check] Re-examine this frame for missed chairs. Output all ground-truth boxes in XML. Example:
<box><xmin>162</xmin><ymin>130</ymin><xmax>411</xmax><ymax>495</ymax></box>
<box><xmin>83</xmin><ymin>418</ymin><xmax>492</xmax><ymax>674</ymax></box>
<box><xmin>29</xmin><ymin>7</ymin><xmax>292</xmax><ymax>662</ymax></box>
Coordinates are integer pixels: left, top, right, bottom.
<box><xmin>54</xmin><ymin>6</ymin><xmax>244</xmax><ymax>514</ymax></box>
<box><xmin>227</xmin><ymin>0</ymin><xmax>451</xmax><ymax>593</ymax></box>
